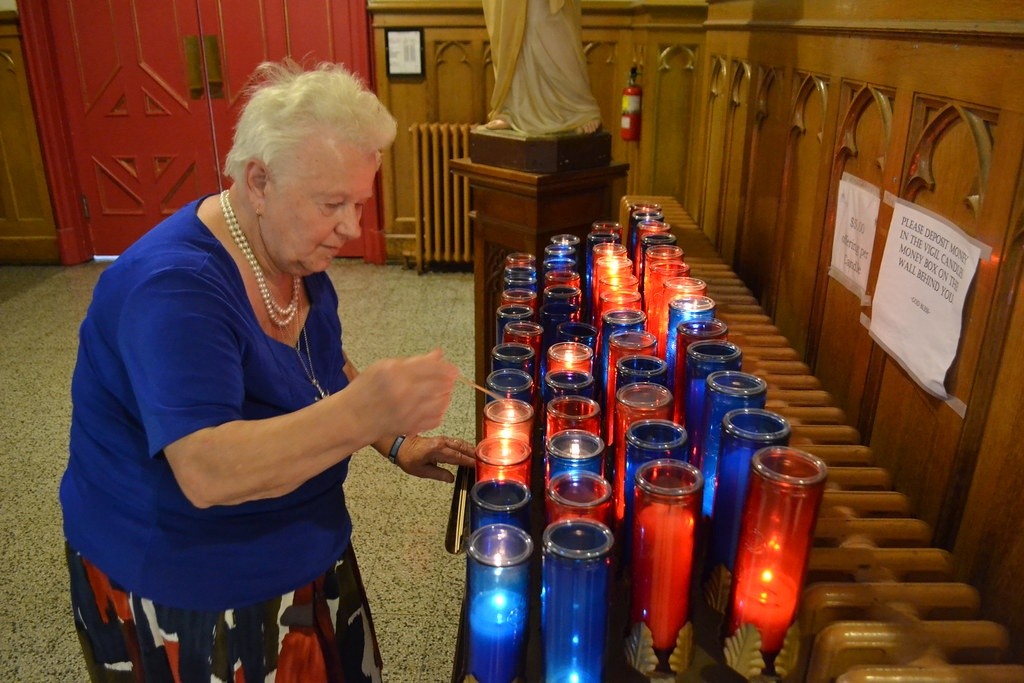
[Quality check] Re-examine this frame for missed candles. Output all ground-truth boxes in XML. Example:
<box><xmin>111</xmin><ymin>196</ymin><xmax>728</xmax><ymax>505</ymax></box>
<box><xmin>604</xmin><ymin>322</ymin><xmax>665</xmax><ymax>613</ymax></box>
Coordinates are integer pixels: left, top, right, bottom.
<box><xmin>463</xmin><ymin>200</ymin><xmax>829</xmax><ymax>683</ymax></box>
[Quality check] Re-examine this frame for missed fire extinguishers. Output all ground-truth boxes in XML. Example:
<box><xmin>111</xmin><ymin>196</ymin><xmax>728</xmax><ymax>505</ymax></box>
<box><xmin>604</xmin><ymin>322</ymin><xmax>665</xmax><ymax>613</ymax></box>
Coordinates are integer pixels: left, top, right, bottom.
<box><xmin>620</xmin><ymin>64</ymin><xmax>641</xmax><ymax>142</ymax></box>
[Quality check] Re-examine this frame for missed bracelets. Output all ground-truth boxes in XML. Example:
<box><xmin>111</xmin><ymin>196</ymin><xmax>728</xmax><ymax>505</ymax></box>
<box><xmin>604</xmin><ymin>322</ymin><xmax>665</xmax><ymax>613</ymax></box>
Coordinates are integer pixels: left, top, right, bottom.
<box><xmin>388</xmin><ymin>435</ymin><xmax>406</xmax><ymax>464</ymax></box>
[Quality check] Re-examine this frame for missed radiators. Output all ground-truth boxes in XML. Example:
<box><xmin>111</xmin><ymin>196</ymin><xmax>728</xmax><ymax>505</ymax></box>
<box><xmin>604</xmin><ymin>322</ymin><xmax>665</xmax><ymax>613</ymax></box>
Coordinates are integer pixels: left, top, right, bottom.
<box><xmin>620</xmin><ymin>196</ymin><xmax>1024</xmax><ymax>683</ymax></box>
<box><xmin>406</xmin><ymin>121</ymin><xmax>473</xmax><ymax>273</ymax></box>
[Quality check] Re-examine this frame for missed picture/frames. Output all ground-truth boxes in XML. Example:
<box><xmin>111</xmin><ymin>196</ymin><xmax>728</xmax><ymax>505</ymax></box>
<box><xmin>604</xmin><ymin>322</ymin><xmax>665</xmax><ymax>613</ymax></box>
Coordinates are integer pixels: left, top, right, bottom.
<box><xmin>383</xmin><ymin>26</ymin><xmax>425</xmax><ymax>77</ymax></box>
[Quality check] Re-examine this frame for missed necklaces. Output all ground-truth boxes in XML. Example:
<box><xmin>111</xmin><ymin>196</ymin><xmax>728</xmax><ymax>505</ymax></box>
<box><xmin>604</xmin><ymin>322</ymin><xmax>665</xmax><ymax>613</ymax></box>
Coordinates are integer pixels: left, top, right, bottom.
<box><xmin>221</xmin><ymin>190</ymin><xmax>330</xmax><ymax>401</ymax></box>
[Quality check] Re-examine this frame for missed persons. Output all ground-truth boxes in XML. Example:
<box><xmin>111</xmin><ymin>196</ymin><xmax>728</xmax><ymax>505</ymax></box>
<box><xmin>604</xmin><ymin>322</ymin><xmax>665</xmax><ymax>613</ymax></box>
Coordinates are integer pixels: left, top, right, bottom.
<box><xmin>481</xmin><ymin>0</ymin><xmax>602</xmax><ymax>135</ymax></box>
<box><xmin>60</xmin><ymin>58</ymin><xmax>474</xmax><ymax>683</ymax></box>
